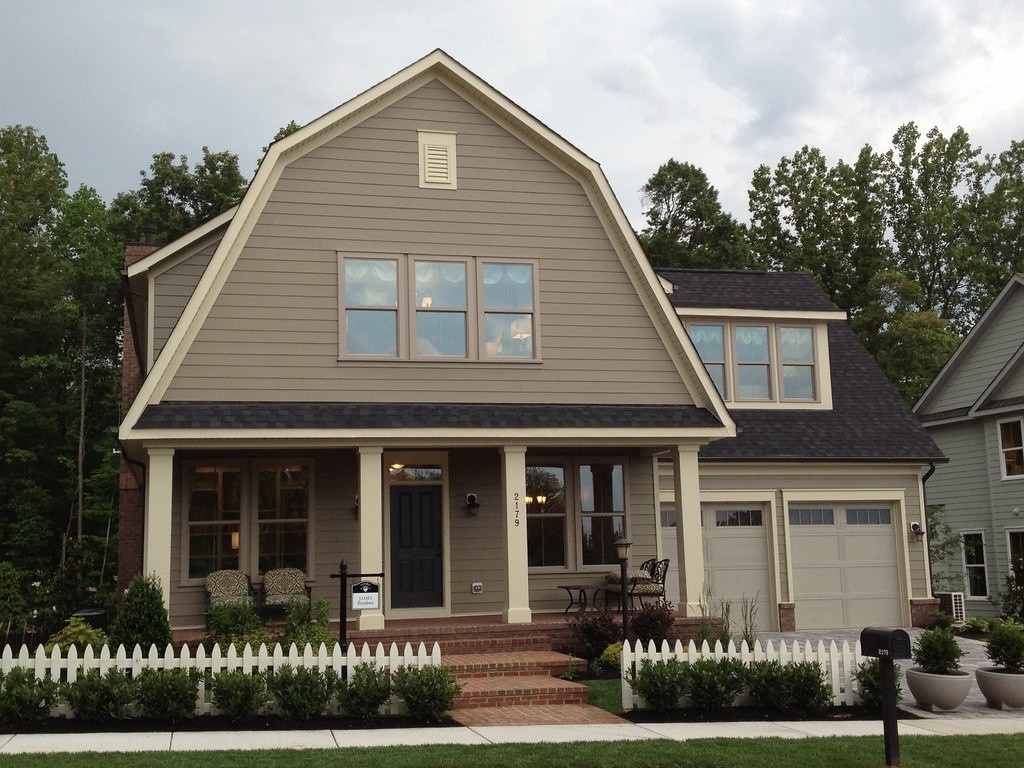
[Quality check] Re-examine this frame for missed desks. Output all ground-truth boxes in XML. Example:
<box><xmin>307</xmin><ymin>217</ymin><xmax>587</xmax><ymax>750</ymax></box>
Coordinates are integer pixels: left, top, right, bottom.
<box><xmin>557</xmin><ymin>584</ymin><xmax>606</xmax><ymax>617</ymax></box>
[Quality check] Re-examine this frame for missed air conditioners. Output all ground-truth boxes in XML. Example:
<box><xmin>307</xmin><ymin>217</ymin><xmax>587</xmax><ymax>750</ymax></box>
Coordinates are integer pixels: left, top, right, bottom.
<box><xmin>932</xmin><ymin>591</ymin><xmax>966</xmax><ymax>624</ymax></box>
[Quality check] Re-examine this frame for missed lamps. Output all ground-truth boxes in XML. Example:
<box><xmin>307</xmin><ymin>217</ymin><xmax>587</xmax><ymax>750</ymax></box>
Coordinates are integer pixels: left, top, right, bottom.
<box><xmin>612</xmin><ymin>535</ymin><xmax>634</xmax><ymax>560</ymax></box>
<box><xmin>910</xmin><ymin>522</ymin><xmax>925</xmax><ymax>541</ymax></box>
<box><xmin>466</xmin><ymin>493</ymin><xmax>481</xmax><ymax>517</ymax></box>
<box><xmin>510</xmin><ymin>318</ymin><xmax>532</xmax><ymax>340</ymax></box>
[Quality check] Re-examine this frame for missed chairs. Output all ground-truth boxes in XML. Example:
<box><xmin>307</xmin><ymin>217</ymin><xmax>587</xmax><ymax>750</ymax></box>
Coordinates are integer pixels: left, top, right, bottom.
<box><xmin>258</xmin><ymin>568</ymin><xmax>313</xmax><ymax>628</ymax></box>
<box><xmin>204</xmin><ymin>570</ymin><xmax>259</xmax><ymax>635</ymax></box>
<box><xmin>603</xmin><ymin>558</ymin><xmax>670</xmax><ymax>612</ymax></box>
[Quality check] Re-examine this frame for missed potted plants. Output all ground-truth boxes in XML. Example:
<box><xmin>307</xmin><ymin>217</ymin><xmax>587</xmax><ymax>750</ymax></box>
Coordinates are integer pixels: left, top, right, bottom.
<box><xmin>906</xmin><ymin>625</ymin><xmax>973</xmax><ymax>713</ymax></box>
<box><xmin>976</xmin><ymin>616</ymin><xmax>1024</xmax><ymax>710</ymax></box>
